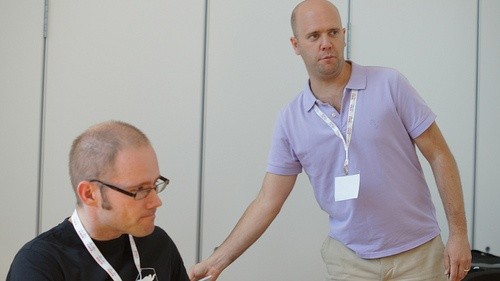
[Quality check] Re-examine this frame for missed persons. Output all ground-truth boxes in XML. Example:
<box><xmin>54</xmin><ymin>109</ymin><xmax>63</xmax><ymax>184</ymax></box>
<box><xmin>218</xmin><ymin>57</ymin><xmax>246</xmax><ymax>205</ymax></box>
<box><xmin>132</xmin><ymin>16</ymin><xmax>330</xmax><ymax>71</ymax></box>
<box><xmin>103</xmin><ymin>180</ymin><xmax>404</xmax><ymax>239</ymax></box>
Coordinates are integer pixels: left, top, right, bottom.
<box><xmin>188</xmin><ymin>0</ymin><xmax>472</xmax><ymax>281</ymax></box>
<box><xmin>5</xmin><ymin>120</ymin><xmax>190</xmax><ymax>281</ymax></box>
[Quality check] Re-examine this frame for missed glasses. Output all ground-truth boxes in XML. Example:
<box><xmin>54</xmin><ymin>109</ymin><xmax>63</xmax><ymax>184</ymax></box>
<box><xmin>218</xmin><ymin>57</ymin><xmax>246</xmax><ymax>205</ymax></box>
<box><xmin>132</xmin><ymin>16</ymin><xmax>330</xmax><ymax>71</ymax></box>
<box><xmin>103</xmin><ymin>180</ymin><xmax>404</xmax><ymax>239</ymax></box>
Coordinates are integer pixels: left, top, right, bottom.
<box><xmin>89</xmin><ymin>176</ymin><xmax>169</xmax><ymax>200</ymax></box>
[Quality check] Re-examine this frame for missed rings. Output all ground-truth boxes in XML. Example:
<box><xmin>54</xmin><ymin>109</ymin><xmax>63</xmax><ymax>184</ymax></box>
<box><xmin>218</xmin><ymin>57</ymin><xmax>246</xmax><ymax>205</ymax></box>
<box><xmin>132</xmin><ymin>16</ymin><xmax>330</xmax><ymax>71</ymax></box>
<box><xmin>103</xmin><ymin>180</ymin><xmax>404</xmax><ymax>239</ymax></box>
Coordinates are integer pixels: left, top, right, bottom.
<box><xmin>463</xmin><ymin>269</ymin><xmax>470</xmax><ymax>272</ymax></box>
